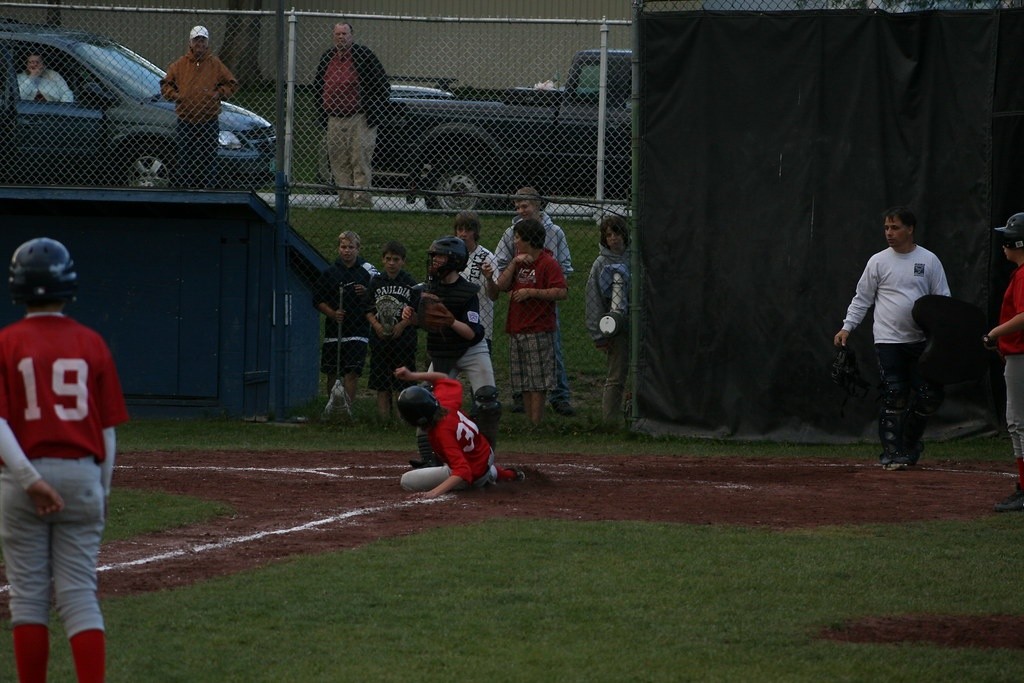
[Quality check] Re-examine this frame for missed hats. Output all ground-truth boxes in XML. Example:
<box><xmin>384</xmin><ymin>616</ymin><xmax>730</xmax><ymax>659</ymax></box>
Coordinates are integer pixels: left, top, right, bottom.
<box><xmin>190</xmin><ymin>26</ymin><xmax>208</xmax><ymax>39</ymax></box>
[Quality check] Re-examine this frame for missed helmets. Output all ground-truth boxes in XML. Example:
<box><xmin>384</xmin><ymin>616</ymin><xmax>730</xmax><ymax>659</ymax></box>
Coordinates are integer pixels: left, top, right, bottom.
<box><xmin>994</xmin><ymin>213</ymin><xmax>1024</xmax><ymax>235</ymax></box>
<box><xmin>8</xmin><ymin>237</ymin><xmax>85</xmax><ymax>306</ymax></box>
<box><xmin>427</xmin><ymin>237</ymin><xmax>468</xmax><ymax>279</ymax></box>
<box><xmin>397</xmin><ymin>386</ymin><xmax>438</xmax><ymax>427</ymax></box>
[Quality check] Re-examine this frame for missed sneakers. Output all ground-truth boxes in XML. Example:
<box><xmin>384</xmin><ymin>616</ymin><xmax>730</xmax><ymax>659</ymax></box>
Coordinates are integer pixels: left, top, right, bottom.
<box><xmin>504</xmin><ymin>467</ymin><xmax>525</xmax><ymax>481</ymax></box>
<box><xmin>883</xmin><ymin>461</ymin><xmax>908</xmax><ymax>470</ymax></box>
<box><xmin>993</xmin><ymin>482</ymin><xmax>1024</xmax><ymax>512</ymax></box>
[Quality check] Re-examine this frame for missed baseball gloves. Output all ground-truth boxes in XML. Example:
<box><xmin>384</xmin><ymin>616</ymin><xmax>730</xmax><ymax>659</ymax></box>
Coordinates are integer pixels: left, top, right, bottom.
<box><xmin>413</xmin><ymin>291</ymin><xmax>456</xmax><ymax>336</ymax></box>
<box><xmin>828</xmin><ymin>343</ymin><xmax>873</xmax><ymax>405</ymax></box>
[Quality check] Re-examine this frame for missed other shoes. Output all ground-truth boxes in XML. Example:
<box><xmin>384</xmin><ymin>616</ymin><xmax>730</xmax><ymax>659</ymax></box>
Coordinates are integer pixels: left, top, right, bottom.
<box><xmin>510</xmin><ymin>404</ymin><xmax>525</xmax><ymax>413</ymax></box>
<box><xmin>336</xmin><ymin>200</ymin><xmax>374</xmax><ymax>207</ymax></box>
<box><xmin>554</xmin><ymin>402</ymin><xmax>577</xmax><ymax>416</ymax></box>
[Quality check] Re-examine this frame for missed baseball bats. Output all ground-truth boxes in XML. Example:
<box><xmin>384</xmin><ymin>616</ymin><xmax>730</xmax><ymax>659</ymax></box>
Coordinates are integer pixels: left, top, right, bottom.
<box><xmin>982</xmin><ymin>334</ymin><xmax>1006</xmax><ymax>364</ymax></box>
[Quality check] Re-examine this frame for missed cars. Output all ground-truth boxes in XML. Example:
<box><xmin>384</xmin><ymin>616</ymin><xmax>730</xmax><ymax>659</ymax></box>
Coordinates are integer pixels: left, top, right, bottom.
<box><xmin>0</xmin><ymin>17</ymin><xmax>277</xmax><ymax>189</ymax></box>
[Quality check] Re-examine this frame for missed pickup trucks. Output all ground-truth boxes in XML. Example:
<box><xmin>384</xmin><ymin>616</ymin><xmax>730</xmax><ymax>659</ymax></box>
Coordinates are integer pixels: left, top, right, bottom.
<box><xmin>327</xmin><ymin>48</ymin><xmax>632</xmax><ymax>218</ymax></box>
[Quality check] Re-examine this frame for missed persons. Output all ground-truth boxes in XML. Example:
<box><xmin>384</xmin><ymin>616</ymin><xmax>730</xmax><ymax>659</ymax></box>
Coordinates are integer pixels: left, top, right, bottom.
<box><xmin>982</xmin><ymin>213</ymin><xmax>1024</xmax><ymax>511</ymax></box>
<box><xmin>401</xmin><ymin>236</ymin><xmax>501</xmax><ymax>469</ymax></box>
<box><xmin>17</xmin><ymin>55</ymin><xmax>74</xmax><ymax>102</ymax></box>
<box><xmin>0</xmin><ymin>239</ymin><xmax>128</xmax><ymax>683</ymax></box>
<box><xmin>312</xmin><ymin>230</ymin><xmax>381</xmax><ymax>402</ymax></box>
<box><xmin>160</xmin><ymin>26</ymin><xmax>236</xmax><ymax>190</ymax></box>
<box><xmin>427</xmin><ymin>210</ymin><xmax>500</xmax><ymax>419</ymax></box>
<box><xmin>366</xmin><ymin>241</ymin><xmax>419</xmax><ymax>419</ymax></box>
<box><xmin>835</xmin><ymin>208</ymin><xmax>952</xmax><ymax>469</ymax></box>
<box><xmin>493</xmin><ymin>186</ymin><xmax>578</xmax><ymax>417</ymax></box>
<box><xmin>497</xmin><ymin>217</ymin><xmax>570</xmax><ymax>424</ymax></box>
<box><xmin>313</xmin><ymin>22</ymin><xmax>391</xmax><ymax>208</ymax></box>
<box><xmin>393</xmin><ymin>366</ymin><xmax>526</xmax><ymax>499</ymax></box>
<box><xmin>584</xmin><ymin>215</ymin><xmax>632</xmax><ymax>428</ymax></box>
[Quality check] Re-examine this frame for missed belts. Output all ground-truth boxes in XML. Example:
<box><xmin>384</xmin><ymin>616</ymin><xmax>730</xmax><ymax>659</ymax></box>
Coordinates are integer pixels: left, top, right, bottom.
<box><xmin>93</xmin><ymin>458</ymin><xmax>100</xmax><ymax>466</ymax></box>
